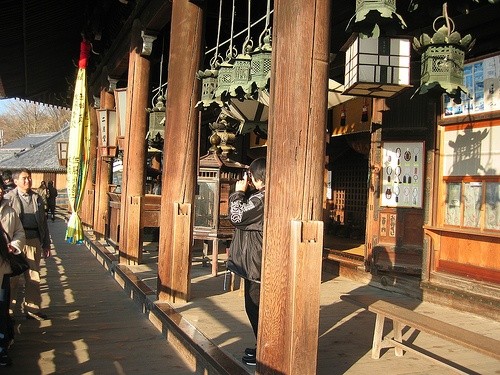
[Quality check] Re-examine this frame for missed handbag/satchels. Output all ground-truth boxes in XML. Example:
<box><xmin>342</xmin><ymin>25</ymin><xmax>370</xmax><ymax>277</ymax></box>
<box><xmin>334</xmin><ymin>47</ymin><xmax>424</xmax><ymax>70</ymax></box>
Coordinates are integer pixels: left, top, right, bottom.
<box><xmin>7</xmin><ymin>251</ymin><xmax>29</xmax><ymax>278</ymax></box>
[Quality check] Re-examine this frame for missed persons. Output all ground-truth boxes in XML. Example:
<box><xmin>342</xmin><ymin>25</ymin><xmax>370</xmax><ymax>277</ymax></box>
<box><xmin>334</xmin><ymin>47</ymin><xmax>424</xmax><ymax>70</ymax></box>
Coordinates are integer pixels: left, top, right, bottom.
<box><xmin>3</xmin><ymin>168</ymin><xmax>51</xmax><ymax>320</ymax></box>
<box><xmin>45</xmin><ymin>180</ymin><xmax>58</xmax><ymax>220</ymax></box>
<box><xmin>0</xmin><ymin>175</ymin><xmax>26</xmax><ymax>366</ymax></box>
<box><xmin>37</xmin><ymin>181</ymin><xmax>50</xmax><ymax>219</ymax></box>
<box><xmin>2</xmin><ymin>170</ymin><xmax>18</xmax><ymax>195</ymax></box>
<box><xmin>226</xmin><ymin>157</ymin><xmax>267</xmax><ymax>365</ymax></box>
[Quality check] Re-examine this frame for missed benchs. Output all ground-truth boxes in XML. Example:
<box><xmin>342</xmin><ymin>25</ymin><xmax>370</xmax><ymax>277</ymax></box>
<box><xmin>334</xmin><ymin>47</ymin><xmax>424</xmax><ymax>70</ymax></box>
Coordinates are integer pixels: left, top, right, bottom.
<box><xmin>340</xmin><ymin>294</ymin><xmax>500</xmax><ymax>375</ymax></box>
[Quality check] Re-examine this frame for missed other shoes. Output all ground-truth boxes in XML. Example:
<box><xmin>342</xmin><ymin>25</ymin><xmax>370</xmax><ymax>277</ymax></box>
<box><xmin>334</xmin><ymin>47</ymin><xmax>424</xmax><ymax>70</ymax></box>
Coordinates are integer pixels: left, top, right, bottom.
<box><xmin>244</xmin><ymin>347</ymin><xmax>256</xmax><ymax>356</ymax></box>
<box><xmin>26</xmin><ymin>312</ymin><xmax>49</xmax><ymax>320</ymax></box>
<box><xmin>242</xmin><ymin>355</ymin><xmax>256</xmax><ymax>366</ymax></box>
<box><xmin>0</xmin><ymin>352</ymin><xmax>10</xmax><ymax>366</ymax></box>
<box><xmin>0</xmin><ymin>335</ymin><xmax>15</xmax><ymax>352</ymax></box>
